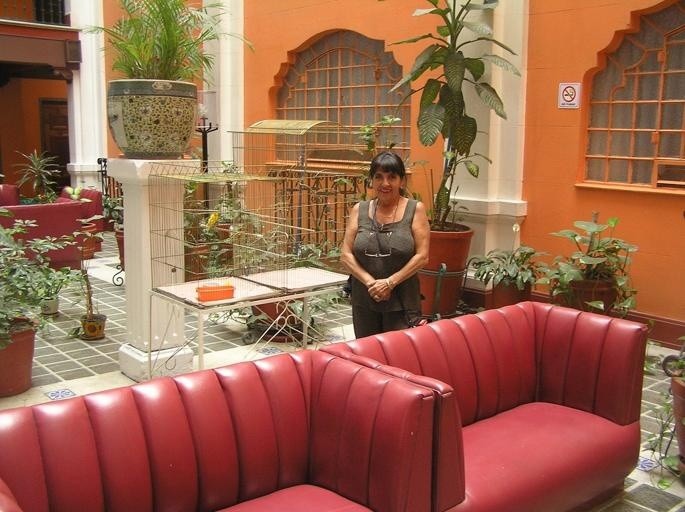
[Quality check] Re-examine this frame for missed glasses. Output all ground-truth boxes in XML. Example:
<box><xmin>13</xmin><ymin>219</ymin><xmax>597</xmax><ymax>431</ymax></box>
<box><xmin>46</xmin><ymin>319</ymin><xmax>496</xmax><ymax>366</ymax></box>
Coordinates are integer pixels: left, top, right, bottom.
<box><xmin>364</xmin><ymin>232</ymin><xmax>393</xmax><ymax>258</ymax></box>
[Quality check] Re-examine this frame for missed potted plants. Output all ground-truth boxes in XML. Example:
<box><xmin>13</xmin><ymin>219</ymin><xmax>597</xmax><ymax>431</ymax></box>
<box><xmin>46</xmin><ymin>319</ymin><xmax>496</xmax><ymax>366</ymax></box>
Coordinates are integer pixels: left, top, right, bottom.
<box><xmin>386</xmin><ymin>0</ymin><xmax>522</xmax><ymax>323</ymax></box>
<box><xmin>81</xmin><ymin>0</ymin><xmax>255</xmax><ymax>159</ymax></box>
<box><xmin>470</xmin><ymin>222</ymin><xmax>549</xmax><ymax>310</ymax></box>
<box><xmin>642</xmin><ymin>335</ymin><xmax>685</xmax><ymax>490</ymax></box>
<box><xmin>549</xmin><ymin>212</ymin><xmax>638</xmax><ymax>319</ymax></box>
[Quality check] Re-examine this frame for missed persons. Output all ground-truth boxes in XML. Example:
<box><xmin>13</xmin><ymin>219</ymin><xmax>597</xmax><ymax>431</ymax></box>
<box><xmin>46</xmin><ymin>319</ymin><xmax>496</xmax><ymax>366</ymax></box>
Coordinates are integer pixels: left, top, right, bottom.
<box><xmin>338</xmin><ymin>149</ymin><xmax>432</xmax><ymax>339</ymax></box>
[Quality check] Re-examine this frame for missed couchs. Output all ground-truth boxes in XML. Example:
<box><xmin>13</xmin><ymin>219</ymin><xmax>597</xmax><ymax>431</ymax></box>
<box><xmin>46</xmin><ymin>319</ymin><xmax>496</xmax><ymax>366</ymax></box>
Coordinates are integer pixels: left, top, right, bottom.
<box><xmin>319</xmin><ymin>301</ymin><xmax>649</xmax><ymax>512</ymax></box>
<box><xmin>0</xmin><ymin>350</ymin><xmax>435</xmax><ymax>512</ymax></box>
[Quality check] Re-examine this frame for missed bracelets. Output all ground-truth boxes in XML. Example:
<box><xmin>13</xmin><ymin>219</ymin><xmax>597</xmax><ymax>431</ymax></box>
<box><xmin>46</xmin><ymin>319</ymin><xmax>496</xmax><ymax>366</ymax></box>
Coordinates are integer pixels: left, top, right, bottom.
<box><xmin>385</xmin><ymin>277</ymin><xmax>394</xmax><ymax>291</ymax></box>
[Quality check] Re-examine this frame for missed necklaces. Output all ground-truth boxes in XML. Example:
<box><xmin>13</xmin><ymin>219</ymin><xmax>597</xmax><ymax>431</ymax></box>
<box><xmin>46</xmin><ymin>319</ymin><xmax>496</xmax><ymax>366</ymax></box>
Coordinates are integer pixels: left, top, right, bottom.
<box><xmin>376</xmin><ymin>203</ymin><xmax>397</xmax><ymax>218</ymax></box>
